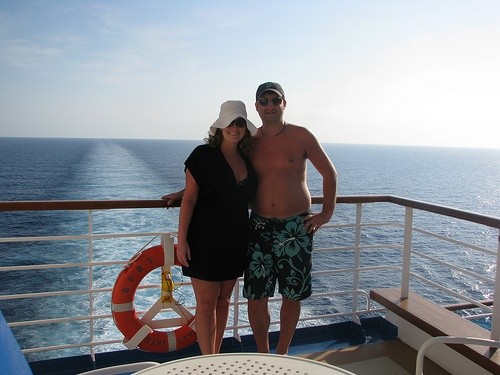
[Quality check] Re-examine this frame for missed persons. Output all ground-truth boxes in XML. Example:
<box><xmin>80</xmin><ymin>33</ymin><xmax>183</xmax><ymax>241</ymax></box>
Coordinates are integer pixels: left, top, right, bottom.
<box><xmin>162</xmin><ymin>82</ymin><xmax>338</xmax><ymax>355</ymax></box>
<box><xmin>177</xmin><ymin>101</ymin><xmax>258</xmax><ymax>355</ymax></box>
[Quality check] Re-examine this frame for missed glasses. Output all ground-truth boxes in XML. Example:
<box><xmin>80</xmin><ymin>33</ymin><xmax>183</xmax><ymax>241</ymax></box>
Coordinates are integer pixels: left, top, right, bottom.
<box><xmin>227</xmin><ymin>119</ymin><xmax>248</xmax><ymax>129</ymax></box>
<box><xmin>258</xmin><ymin>98</ymin><xmax>283</xmax><ymax>106</ymax></box>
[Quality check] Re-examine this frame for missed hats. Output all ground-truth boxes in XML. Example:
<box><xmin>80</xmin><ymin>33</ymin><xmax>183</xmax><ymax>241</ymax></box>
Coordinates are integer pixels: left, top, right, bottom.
<box><xmin>256</xmin><ymin>82</ymin><xmax>285</xmax><ymax>99</ymax></box>
<box><xmin>208</xmin><ymin>100</ymin><xmax>258</xmax><ymax>137</ymax></box>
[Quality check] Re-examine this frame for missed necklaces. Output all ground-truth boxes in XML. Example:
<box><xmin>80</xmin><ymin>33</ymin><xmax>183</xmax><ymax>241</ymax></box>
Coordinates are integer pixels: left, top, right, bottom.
<box><xmin>261</xmin><ymin>121</ymin><xmax>285</xmax><ymax>136</ymax></box>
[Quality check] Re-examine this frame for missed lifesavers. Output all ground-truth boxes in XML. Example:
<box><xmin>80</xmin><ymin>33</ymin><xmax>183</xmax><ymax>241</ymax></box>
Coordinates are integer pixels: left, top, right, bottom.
<box><xmin>110</xmin><ymin>243</ymin><xmax>200</xmax><ymax>353</ymax></box>
